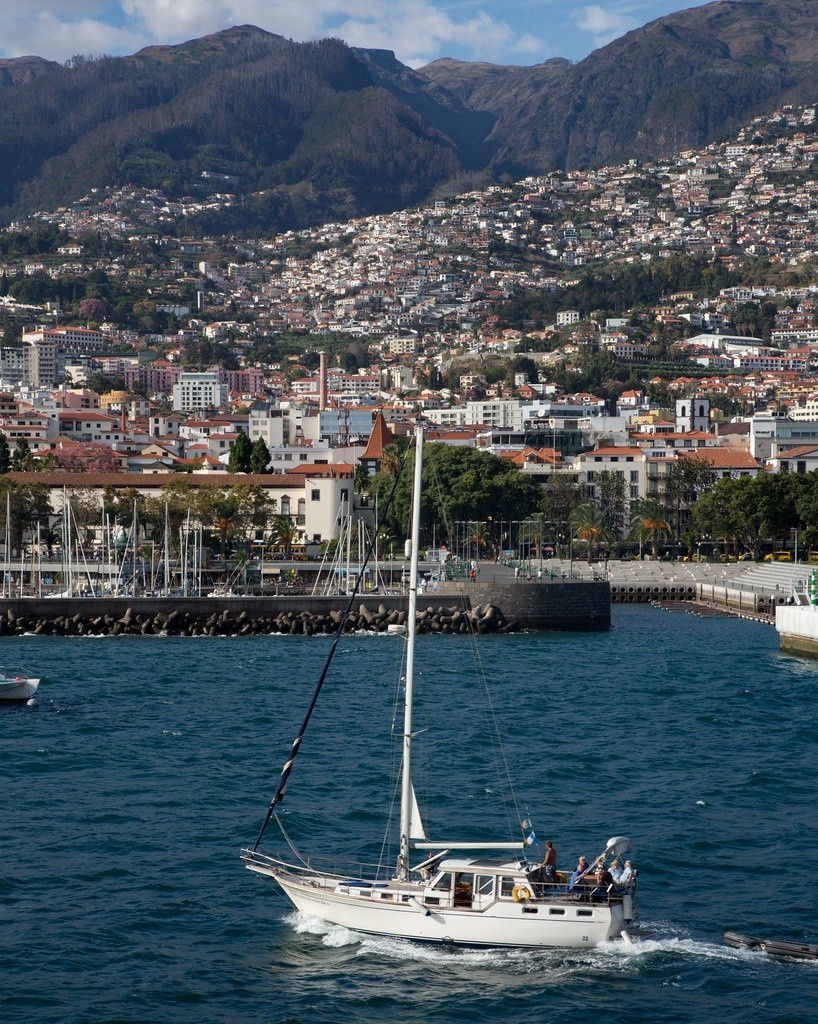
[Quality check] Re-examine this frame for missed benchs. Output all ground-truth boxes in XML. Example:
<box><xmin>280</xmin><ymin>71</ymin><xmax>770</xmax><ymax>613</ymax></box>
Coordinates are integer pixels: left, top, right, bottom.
<box><xmin>541</xmin><ymin>884</ymin><xmax>631</xmax><ymax>904</ymax></box>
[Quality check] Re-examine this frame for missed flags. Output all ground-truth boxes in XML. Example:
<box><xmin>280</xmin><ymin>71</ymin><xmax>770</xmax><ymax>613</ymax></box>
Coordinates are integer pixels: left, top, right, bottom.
<box><xmin>526</xmin><ymin>832</ymin><xmax>536</xmax><ymax>845</ymax></box>
<box><xmin>522</xmin><ymin>819</ymin><xmax>533</xmax><ymax>829</ymax></box>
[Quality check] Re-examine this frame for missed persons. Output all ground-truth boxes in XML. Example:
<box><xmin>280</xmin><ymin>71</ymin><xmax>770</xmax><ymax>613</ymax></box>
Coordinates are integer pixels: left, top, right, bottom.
<box><xmin>538</xmin><ymin>568</ymin><xmax>542</xmax><ymax>583</ymax></box>
<box><xmin>515</xmin><ymin>566</ymin><xmax>520</xmax><ymax>579</ymax></box>
<box><xmin>541</xmin><ymin>841</ymin><xmax>557</xmax><ymax>888</ymax></box>
<box><xmin>569</xmin><ymin>856</ymin><xmax>634</xmax><ymax>901</ymax></box>
<box><xmin>468</xmin><ymin>559</ymin><xmax>477</xmax><ymax>583</ymax></box>
<box><xmin>527</xmin><ymin>572</ymin><xmax>531</xmax><ymax>583</ymax></box>
<box><xmin>427</xmin><ymin>853</ymin><xmax>434</xmax><ymax>876</ymax></box>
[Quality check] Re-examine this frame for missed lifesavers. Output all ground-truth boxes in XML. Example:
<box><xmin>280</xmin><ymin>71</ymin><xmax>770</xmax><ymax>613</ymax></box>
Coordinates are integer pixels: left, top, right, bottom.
<box><xmin>511</xmin><ymin>884</ymin><xmax>531</xmax><ymax>904</ymax></box>
<box><xmin>554</xmin><ymin>871</ymin><xmax>567</xmax><ymax>884</ymax></box>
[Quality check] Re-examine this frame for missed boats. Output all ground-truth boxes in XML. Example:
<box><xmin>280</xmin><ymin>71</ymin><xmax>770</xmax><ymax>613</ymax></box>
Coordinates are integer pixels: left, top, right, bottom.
<box><xmin>0</xmin><ymin>666</ymin><xmax>40</xmax><ymax>700</ymax></box>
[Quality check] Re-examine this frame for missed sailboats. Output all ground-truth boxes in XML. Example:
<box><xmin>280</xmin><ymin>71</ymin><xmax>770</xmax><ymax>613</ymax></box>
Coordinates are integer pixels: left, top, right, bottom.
<box><xmin>238</xmin><ymin>426</ymin><xmax>633</xmax><ymax>943</ymax></box>
<box><xmin>3</xmin><ymin>483</ymin><xmax>408</xmax><ymax>597</ymax></box>
